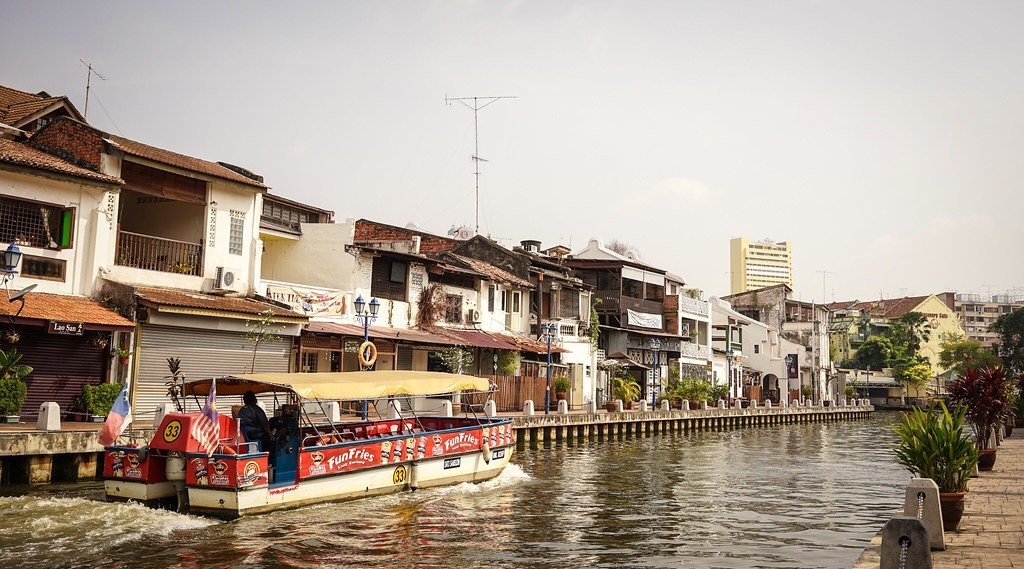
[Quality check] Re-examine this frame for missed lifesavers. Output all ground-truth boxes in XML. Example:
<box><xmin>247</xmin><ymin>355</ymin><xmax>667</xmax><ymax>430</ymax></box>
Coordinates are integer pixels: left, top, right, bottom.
<box><xmin>359</xmin><ymin>341</ymin><xmax>377</xmax><ymax>366</ymax></box>
<box><xmin>315</xmin><ymin>435</ymin><xmax>339</xmax><ymax>446</ymax></box>
<box><xmin>217</xmin><ymin>446</ymin><xmax>236</xmax><ymax>454</ymax></box>
<box><xmin>127</xmin><ymin>444</ymin><xmax>138</xmax><ymax>448</ymax></box>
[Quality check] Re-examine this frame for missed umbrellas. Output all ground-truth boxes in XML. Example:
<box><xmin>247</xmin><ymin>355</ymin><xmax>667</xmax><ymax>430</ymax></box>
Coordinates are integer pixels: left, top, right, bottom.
<box><xmin>597</xmin><ymin>351</ymin><xmax>653</xmax><ymax>371</ymax></box>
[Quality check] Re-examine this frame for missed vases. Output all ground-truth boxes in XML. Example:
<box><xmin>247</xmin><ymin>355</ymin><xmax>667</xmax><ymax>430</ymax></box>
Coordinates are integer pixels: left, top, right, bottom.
<box><xmin>606</xmin><ymin>400</ymin><xmax>618</xmax><ymax>412</ymax></box>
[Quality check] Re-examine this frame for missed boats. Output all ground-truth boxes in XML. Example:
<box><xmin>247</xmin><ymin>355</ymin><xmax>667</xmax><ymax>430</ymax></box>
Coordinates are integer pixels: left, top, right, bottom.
<box><xmin>99</xmin><ymin>369</ymin><xmax>516</xmax><ymax>522</ymax></box>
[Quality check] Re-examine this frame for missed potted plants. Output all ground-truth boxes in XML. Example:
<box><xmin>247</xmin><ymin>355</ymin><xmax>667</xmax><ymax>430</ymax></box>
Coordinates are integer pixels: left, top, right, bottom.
<box><xmin>119</xmin><ymin>349</ymin><xmax>134</xmax><ymax>366</ymax></box>
<box><xmin>946</xmin><ymin>367</ymin><xmax>1020</xmax><ymax>471</ymax></box>
<box><xmin>553</xmin><ymin>376</ymin><xmax>570</xmax><ymax>400</ymax></box>
<box><xmin>889</xmin><ymin>398</ymin><xmax>984</xmax><ymax>532</ymax></box>
<box><xmin>0</xmin><ymin>378</ymin><xmax>27</xmax><ymax>423</ymax></box>
<box><xmin>71</xmin><ymin>383</ymin><xmax>124</xmax><ymax>422</ymax></box>
<box><xmin>683</xmin><ymin>379</ymin><xmax>708</xmax><ymax>410</ymax></box>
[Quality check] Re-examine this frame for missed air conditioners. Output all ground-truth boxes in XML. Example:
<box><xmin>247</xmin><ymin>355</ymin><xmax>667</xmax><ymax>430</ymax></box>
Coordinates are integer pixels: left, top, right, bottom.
<box><xmin>528</xmin><ymin>245</ymin><xmax>538</xmax><ymax>252</ymax></box>
<box><xmin>214</xmin><ymin>266</ymin><xmax>242</xmax><ymax>292</ymax></box>
<box><xmin>468</xmin><ymin>309</ymin><xmax>482</xmax><ymax>323</ymax></box>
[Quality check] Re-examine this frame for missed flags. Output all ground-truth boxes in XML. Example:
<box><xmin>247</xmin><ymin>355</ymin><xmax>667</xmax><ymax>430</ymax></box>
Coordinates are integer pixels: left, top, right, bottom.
<box><xmin>190</xmin><ymin>378</ymin><xmax>222</xmax><ymax>457</ymax></box>
<box><xmin>96</xmin><ymin>380</ymin><xmax>132</xmax><ymax>446</ymax></box>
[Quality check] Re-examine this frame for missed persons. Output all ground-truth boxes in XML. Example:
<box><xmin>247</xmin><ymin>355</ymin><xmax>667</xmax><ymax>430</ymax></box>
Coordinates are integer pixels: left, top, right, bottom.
<box><xmin>238</xmin><ymin>390</ymin><xmax>277</xmax><ymax>466</ymax></box>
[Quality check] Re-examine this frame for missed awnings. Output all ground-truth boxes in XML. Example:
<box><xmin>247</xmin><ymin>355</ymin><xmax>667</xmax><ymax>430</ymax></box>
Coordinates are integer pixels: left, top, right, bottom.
<box><xmin>304</xmin><ymin>321</ymin><xmax>567</xmax><ymax>355</ymax></box>
